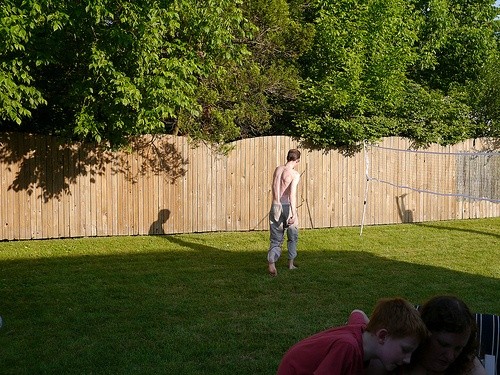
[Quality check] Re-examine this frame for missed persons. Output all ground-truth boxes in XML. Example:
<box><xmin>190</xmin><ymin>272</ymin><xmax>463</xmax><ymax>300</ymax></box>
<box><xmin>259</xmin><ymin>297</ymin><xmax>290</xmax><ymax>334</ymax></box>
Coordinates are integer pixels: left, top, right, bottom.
<box><xmin>276</xmin><ymin>296</ymin><xmax>432</xmax><ymax>375</ymax></box>
<box><xmin>367</xmin><ymin>295</ymin><xmax>480</xmax><ymax>375</ymax></box>
<box><xmin>267</xmin><ymin>149</ymin><xmax>301</xmax><ymax>276</ymax></box>
